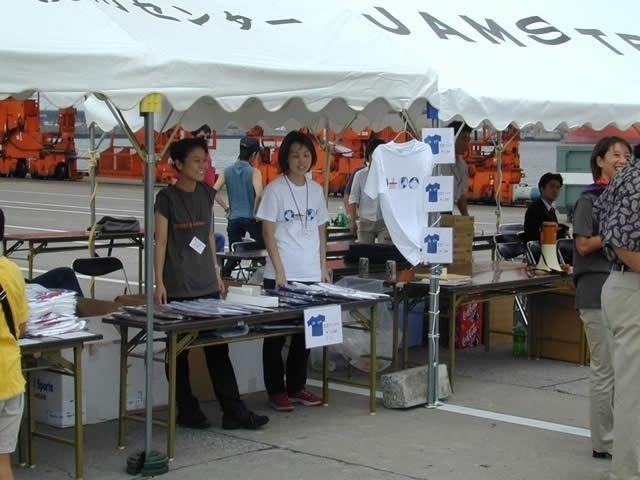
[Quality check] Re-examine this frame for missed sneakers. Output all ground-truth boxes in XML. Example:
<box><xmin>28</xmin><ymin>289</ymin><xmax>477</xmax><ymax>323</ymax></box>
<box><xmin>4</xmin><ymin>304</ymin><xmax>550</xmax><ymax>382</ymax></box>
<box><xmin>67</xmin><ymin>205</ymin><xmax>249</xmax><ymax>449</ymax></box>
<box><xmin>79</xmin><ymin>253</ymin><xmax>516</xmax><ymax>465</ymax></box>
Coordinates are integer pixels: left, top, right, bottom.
<box><xmin>288</xmin><ymin>390</ymin><xmax>324</xmax><ymax>407</ymax></box>
<box><xmin>592</xmin><ymin>450</ymin><xmax>612</xmax><ymax>459</ymax></box>
<box><xmin>222</xmin><ymin>410</ymin><xmax>270</xmax><ymax>430</ymax></box>
<box><xmin>270</xmin><ymin>394</ymin><xmax>296</xmax><ymax>411</ymax></box>
<box><xmin>176</xmin><ymin>407</ymin><xmax>212</xmax><ymax>430</ymax></box>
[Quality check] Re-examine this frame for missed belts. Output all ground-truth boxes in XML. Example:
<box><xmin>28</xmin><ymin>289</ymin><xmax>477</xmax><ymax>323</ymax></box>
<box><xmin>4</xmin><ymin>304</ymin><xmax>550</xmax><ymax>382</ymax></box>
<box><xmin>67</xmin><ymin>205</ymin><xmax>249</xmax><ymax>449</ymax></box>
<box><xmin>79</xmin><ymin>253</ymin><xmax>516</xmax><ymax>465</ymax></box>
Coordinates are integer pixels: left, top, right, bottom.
<box><xmin>612</xmin><ymin>264</ymin><xmax>633</xmax><ymax>272</ymax></box>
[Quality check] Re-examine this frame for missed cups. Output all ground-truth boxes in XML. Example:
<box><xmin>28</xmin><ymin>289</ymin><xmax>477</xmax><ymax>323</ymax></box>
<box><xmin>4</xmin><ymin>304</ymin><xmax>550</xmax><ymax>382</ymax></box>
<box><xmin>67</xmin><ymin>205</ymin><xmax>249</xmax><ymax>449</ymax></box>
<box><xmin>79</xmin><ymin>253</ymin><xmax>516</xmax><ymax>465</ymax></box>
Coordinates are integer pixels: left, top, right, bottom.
<box><xmin>398</xmin><ymin>271</ymin><xmax>415</xmax><ymax>282</ymax></box>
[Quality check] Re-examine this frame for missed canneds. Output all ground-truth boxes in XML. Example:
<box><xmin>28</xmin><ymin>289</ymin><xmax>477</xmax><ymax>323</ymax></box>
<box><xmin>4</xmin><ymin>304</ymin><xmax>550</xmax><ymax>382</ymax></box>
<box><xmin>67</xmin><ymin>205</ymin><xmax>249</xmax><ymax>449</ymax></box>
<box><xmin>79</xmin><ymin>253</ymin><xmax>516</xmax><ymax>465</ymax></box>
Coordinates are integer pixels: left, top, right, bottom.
<box><xmin>359</xmin><ymin>257</ymin><xmax>369</xmax><ymax>279</ymax></box>
<box><xmin>385</xmin><ymin>260</ymin><xmax>397</xmax><ymax>282</ymax></box>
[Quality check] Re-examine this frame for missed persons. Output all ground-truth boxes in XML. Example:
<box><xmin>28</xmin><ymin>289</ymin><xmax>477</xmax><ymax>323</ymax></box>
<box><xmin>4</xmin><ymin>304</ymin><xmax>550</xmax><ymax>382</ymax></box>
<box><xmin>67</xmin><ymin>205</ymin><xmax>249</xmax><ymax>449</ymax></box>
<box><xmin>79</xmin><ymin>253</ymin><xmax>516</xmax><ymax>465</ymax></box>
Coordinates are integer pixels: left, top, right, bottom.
<box><xmin>212</xmin><ymin>138</ymin><xmax>267</xmax><ymax>275</ymax></box>
<box><xmin>429</xmin><ymin>119</ymin><xmax>470</xmax><ymax>231</ymax></box>
<box><xmin>350</xmin><ymin>138</ymin><xmax>397</xmax><ymax>245</ymax></box>
<box><xmin>0</xmin><ymin>209</ymin><xmax>30</xmax><ymax>480</ymax></box>
<box><xmin>253</xmin><ymin>131</ymin><xmax>333</xmax><ymax>412</ymax></box>
<box><xmin>569</xmin><ymin>137</ymin><xmax>632</xmax><ymax>457</ymax></box>
<box><xmin>343</xmin><ymin>160</ymin><xmax>367</xmax><ymax>216</ymax></box>
<box><xmin>153</xmin><ymin>137</ymin><xmax>270</xmax><ymax>432</ymax></box>
<box><xmin>163</xmin><ymin>120</ymin><xmax>220</xmax><ymax>192</ymax></box>
<box><xmin>590</xmin><ymin>143</ymin><xmax>639</xmax><ymax>479</ymax></box>
<box><xmin>526</xmin><ymin>171</ymin><xmax>569</xmax><ymax>264</ymax></box>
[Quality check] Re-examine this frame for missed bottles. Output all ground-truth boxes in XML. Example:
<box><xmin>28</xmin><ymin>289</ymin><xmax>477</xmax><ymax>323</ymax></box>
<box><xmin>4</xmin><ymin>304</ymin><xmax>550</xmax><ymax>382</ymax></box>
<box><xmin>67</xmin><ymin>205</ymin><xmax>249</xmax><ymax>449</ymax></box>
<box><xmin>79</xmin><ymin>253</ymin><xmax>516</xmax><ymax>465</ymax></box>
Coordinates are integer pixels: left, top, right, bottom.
<box><xmin>337</xmin><ymin>205</ymin><xmax>345</xmax><ymax>227</ymax></box>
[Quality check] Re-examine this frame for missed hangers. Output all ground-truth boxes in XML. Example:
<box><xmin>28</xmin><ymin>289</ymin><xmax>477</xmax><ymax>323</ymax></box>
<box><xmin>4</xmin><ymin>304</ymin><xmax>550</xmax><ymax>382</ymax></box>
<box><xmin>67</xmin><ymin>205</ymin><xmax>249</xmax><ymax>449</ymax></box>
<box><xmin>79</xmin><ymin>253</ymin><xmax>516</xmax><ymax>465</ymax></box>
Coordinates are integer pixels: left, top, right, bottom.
<box><xmin>392</xmin><ymin>109</ymin><xmax>417</xmax><ymax>143</ymax></box>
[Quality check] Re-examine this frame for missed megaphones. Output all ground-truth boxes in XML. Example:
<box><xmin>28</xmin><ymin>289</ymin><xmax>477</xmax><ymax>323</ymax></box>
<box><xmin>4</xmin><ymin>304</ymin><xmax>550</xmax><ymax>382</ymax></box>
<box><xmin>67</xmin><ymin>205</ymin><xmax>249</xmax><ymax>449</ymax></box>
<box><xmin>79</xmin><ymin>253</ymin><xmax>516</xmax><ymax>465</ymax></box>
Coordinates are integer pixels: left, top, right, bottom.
<box><xmin>532</xmin><ymin>222</ymin><xmax>564</xmax><ymax>274</ymax></box>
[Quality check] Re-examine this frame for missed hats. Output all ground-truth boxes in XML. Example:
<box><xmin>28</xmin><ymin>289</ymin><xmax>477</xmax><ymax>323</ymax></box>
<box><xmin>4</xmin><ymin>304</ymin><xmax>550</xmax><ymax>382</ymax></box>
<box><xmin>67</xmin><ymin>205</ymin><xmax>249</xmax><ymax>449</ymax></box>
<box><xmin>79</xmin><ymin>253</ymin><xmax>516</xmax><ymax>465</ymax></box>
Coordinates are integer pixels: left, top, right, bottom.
<box><xmin>240</xmin><ymin>136</ymin><xmax>260</xmax><ymax>150</ymax></box>
